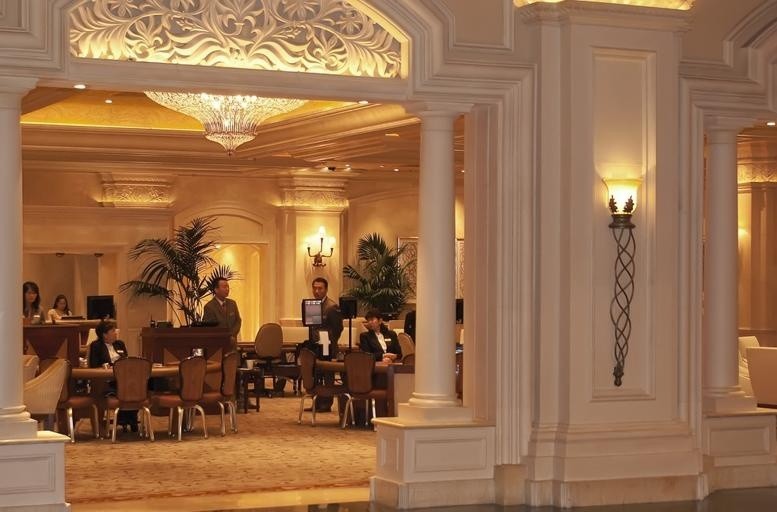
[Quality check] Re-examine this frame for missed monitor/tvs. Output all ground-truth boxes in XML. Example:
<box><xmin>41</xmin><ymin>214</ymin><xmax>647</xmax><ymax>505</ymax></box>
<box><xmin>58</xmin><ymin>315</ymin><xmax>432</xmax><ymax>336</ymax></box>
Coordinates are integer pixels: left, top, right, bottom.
<box><xmin>301</xmin><ymin>299</ymin><xmax>324</xmax><ymax>327</ymax></box>
<box><xmin>339</xmin><ymin>296</ymin><xmax>358</xmax><ymax>319</ymax></box>
<box><xmin>87</xmin><ymin>296</ymin><xmax>114</xmax><ymax>320</ymax></box>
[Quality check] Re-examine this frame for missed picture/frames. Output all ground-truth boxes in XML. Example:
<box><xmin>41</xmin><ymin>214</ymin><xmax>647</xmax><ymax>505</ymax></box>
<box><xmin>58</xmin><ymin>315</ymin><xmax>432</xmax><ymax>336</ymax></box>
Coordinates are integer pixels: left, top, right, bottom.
<box><xmin>395</xmin><ymin>234</ymin><xmax>466</xmax><ymax>306</ymax></box>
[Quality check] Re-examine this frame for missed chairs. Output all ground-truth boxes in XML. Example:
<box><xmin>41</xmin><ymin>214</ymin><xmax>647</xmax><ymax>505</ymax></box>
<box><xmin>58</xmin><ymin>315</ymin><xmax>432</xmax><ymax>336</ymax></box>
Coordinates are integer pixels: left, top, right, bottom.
<box><xmin>17</xmin><ymin>349</ymin><xmax>241</xmax><ymax>444</ymax></box>
<box><xmin>236</xmin><ymin>321</ymin><xmax>464</xmax><ymax>431</ymax></box>
<box><xmin>734</xmin><ymin>334</ymin><xmax>776</xmax><ymax>408</ymax></box>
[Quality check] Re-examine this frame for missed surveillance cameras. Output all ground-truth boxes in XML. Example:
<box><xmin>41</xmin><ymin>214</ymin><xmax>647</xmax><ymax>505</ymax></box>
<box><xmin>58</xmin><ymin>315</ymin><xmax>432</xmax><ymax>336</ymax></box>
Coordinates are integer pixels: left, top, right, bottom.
<box><xmin>55</xmin><ymin>253</ymin><xmax>65</xmax><ymax>257</ymax></box>
<box><xmin>327</xmin><ymin>167</ymin><xmax>336</xmax><ymax>172</ymax></box>
<box><xmin>94</xmin><ymin>251</ymin><xmax>104</xmax><ymax>257</ymax></box>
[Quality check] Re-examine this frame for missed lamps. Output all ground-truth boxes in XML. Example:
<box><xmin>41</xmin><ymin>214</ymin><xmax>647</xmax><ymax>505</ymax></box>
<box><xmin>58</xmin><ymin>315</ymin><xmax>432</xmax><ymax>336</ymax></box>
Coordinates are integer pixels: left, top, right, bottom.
<box><xmin>596</xmin><ymin>173</ymin><xmax>644</xmax><ymax>390</ymax></box>
<box><xmin>304</xmin><ymin>227</ymin><xmax>336</xmax><ymax>269</ymax></box>
<box><xmin>145</xmin><ymin>90</ymin><xmax>312</xmax><ymax>158</ymax></box>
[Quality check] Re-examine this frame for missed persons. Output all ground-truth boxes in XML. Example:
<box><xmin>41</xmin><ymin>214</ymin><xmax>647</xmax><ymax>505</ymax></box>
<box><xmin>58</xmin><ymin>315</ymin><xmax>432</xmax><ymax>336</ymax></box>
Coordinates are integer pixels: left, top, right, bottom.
<box><xmin>87</xmin><ymin>320</ymin><xmax>129</xmax><ymax>367</ymax></box>
<box><xmin>46</xmin><ymin>295</ymin><xmax>73</xmax><ymax>322</ymax></box>
<box><xmin>202</xmin><ymin>275</ymin><xmax>260</xmax><ymax>410</ymax></box>
<box><xmin>24</xmin><ymin>281</ymin><xmax>46</xmax><ymax>324</ymax></box>
<box><xmin>360</xmin><ymin>308</ymin><xmax>402</xmax><ymax>362</ymax></box>
<box><xmin>303</xmin><ymin>277</ymin><xmax>344</xmax><ymax>413</ymax></box>
